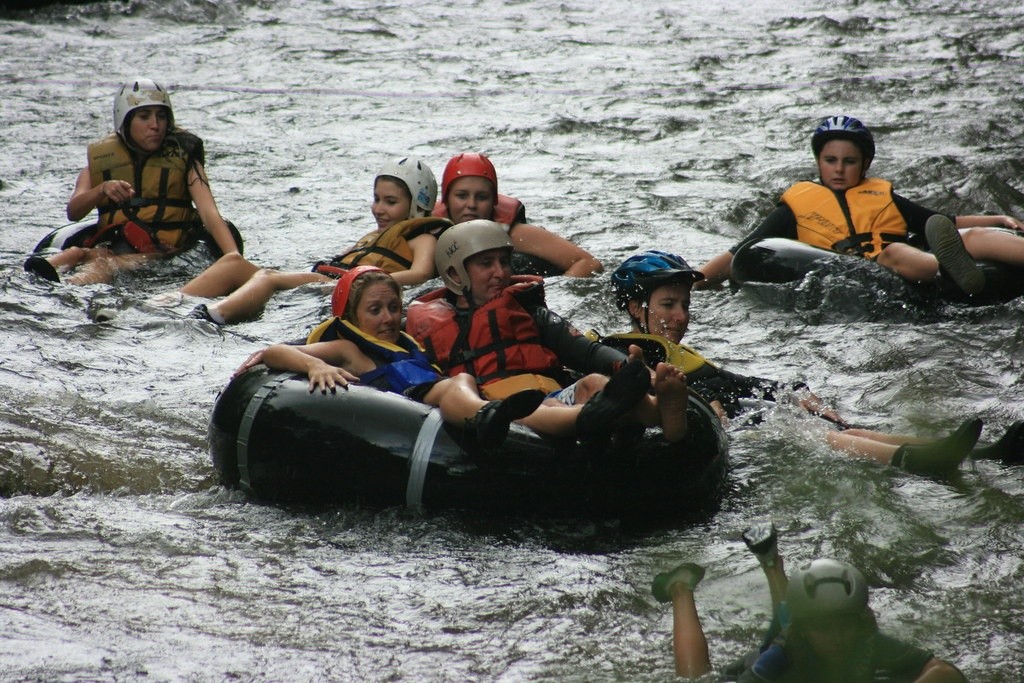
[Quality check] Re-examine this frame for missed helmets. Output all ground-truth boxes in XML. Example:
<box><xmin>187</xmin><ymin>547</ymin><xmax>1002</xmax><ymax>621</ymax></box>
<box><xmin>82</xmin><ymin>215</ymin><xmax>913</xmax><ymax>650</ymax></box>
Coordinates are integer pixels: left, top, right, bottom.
<box><xmin>372</xmin><ymin>157</ymin><xmax>438</xmax><ymax>221</ymax></box>
<box><xmin>331</xmin><ymin>265</ymin><xmax>395</xmax><ymax>325</ymax></box>
<box><xmin>435</xmin><ymin>219</ymin><xmax>515</xmax><ymax>297</ymax></box>
<box><xmin>811</xmin><ymin>115</ymin><xmax>876</xmax><ymax>166</ymax></box>
<box><xmin>785</xmin><ymin>556</ymin><xmax>868</xmax><ymax>618</ymax></box>
<box><xmin>610</xmin><ymin>248</ymin><xmax>705</xmax><ymax>312</ymax></box>
<box><xmin>109</xmin><ymin>78</ymin><xmax>174</xmax><ymax>137</ymax></box>
<box><xmin>441</xmin><ymin>152</ymin><xmax>498</xmax><ymax>206</ymax></box>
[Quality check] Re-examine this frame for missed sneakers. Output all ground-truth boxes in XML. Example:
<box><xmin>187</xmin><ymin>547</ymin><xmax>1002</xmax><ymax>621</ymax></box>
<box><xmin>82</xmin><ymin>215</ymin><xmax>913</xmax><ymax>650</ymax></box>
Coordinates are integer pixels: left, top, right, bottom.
<box><xmin>179</xmin><ymin>303</ymin><xmax>225</xmax><ymax>329</ymax></box>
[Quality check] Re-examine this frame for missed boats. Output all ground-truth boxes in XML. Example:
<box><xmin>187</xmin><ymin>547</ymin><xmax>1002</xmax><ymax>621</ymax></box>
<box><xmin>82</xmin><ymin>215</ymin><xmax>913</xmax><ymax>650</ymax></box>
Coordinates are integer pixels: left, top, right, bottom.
<box><xmin>30</xmin><ymin>212</ymin><xmax>246</xmax><ymax>298</ymax></box>
<box><xmin>732</xmin><ymin>237</ymin><xmax>1024</xmax><ymax>322</ymax></box>
<box><xmin>205</xmin><ymin>334</ymin><xmax>737</xmax><ymax>546</ymax></box>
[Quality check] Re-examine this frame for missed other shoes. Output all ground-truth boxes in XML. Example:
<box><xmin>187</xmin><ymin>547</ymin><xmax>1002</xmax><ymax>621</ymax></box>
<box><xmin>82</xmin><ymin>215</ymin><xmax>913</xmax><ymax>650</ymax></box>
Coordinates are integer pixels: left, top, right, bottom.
<box><xmin>968</xmin><ymin>418</ymin><xmax>1024</xmax><ymax>468</ymax></box>
<box><xmin>739</xmin><ymin>517</ymin><xmax>778</xmax><ymax>552</ymax></box>
<box><xmin>576</xmin><ymin>363</ymin><xmax>651</xmax><ymax>446</ymax></box>
<box><xmin>924</xmin><ymin>215</ymin><xmax>985</xmax><ymax>300</ymax></box>
<box><xmin>464</xmin><ymin>388</ymin><xmax>546</xmax><ymax>444</ymax></box>
<box><xmin>650</xmin><ymin>562</ymin><xmax>707</xmax><ymax>604</ymax></box>
<box><xmin>890</xmin><ymin>417</ymin><xmax>984</xmax><ymax>485</ymax></box>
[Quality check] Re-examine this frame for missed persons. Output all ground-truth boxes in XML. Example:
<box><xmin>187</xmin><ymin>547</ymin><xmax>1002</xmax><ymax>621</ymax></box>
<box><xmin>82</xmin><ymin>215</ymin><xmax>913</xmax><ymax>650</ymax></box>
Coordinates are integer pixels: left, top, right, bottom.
<box><xmin>233</xmin><ymin>219</ymin><xmax>689</xmax><ymax>443</ymax></box>
<box><xmin>95</xmin><ymin>159</ymin><xmax>455</xmax><ymax>325</ymax></box>
<box><xmin>262</xmin><ymin>264</ymin><xmax>650</xmax><ymax>449</ymax></box>
<box><xmin>587</xmin><ymin>250</ymin><xmax>1024</xmax><ymax>476</ymax></box>
<box><xmin>693</xmin><ymin>115</ymin><xmax>1024</xmax><ymax>298</ymax></box>
<box><xmin>24</xmin><ymin>78</ymin><xmax>238</xmax><ymax>283</ymax></box>
<box><xmin>652</xmin><ymin>523</ymin><xmax>968</xmax><ymax>683</ymax></box>
<box><xmin>431</xmin><ymin>153</ymin><xmax>604</xmax><ymax>277</ymax></box>
